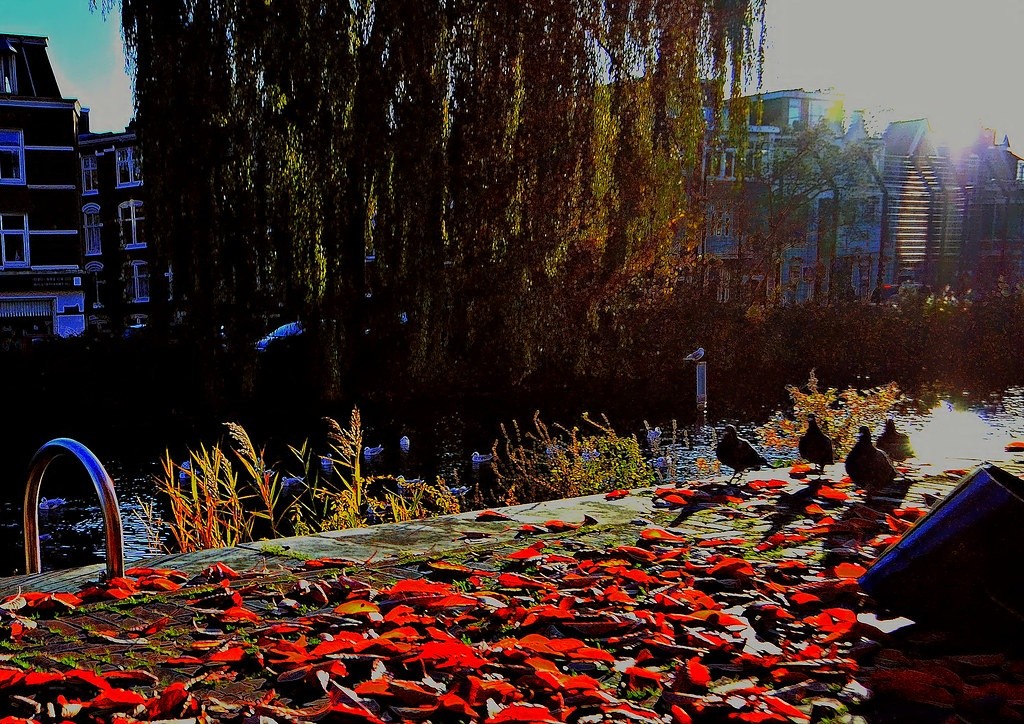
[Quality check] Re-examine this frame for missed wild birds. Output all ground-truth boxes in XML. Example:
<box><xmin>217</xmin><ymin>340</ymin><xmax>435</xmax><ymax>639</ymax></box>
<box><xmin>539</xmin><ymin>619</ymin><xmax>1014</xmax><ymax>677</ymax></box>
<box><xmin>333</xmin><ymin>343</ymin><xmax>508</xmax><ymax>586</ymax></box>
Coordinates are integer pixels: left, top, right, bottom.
<box><xmin>682</xmin><ymin>347</ymin><xmax>706</xmax><ymax>365</ymax></box>
<box><xmin>845</xmin><ymin>418</ymin><xmax>920</xmax><ymax>496</ymax></box>
<box><xmin>396</xmin><ymin>475</ymin><xmax>425</xmax><ymax>489</ymax></box>
<box><xmin>364</xmin><ymin>444</ymin><xmax>384</xmax><ymax>456</ymax></box>
<box><xmin>444</xmin><ymin>485</ymin><xmax>471</xmax><ymax>496</ymax></box>
<box><xmin>281</xmin><ymin>477</ymin><xmax>306</xmax><ymax>486</ymax></box>
<box><xmin>179</xmin><ymin>460</ymin><xmax>201</xmax><ymax>478</ymax></box>
<box><xmin>471</xmin><ymin>451</ymin><xmax>493</xmax><ymax>462</ymax></box>
<box><xmin>320</xmin><ymin>453</ymin><xmax>334</xmax><ymax>465</ymax></box>
<box><xmin>39</xmin><ymin>497</ymin><xmax>67</xmax><ymax>510</ymax></box>
<box><xmin>715</xmin><ymin>425</ymin><xmax>778</xmax><ymax>487</ymax></box>
<box><xmin>400</xmin><ymin>436</ymin><xmax>409</xmax><ymax>446</ymax></box>
<box><xmin>799</xmin><ymin>412</ymin><xmax>835</xmax><ymax>475</ymax></box>
<box><xmin>545</xmin><ymin>426</ymin><xmax>672</xmax><ymax>469</ymax></box>
